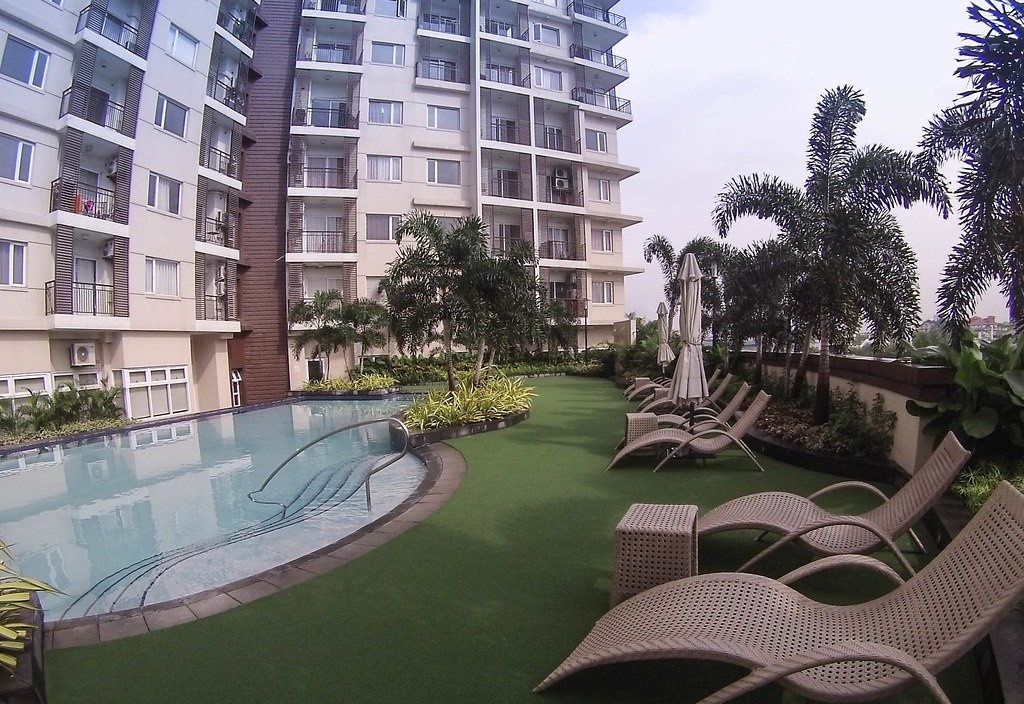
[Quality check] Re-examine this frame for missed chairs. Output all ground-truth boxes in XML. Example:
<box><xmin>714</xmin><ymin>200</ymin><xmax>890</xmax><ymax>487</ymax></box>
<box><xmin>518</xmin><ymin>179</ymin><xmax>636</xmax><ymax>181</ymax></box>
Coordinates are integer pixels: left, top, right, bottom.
<box><xmin>535</xmin><ymin>429</ymin><xmax>1024</xmax><ymax>704</ymax></box>
<box><xmin>603</xmin><ymin>370</ymin><xmax>772</xmax><ymax>473</ymax></box>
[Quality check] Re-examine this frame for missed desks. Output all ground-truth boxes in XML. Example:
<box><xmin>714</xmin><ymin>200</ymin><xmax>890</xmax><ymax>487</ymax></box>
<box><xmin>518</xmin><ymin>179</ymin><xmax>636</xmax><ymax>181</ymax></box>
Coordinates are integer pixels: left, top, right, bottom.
<box><xmin>625</xmin><ymin>413</ymin><xmax>659</xmax><ymax>447</ymax></box>
<box><xmin>635</xmin><ymin>378</ymin><xmax>651</xmax><ymax>395</ymax></box>
<box><xmin>206</xmin><ymin>231</ymin><xmax>220</xmax><ymax>243</ymax></box>
<box><xmin>608</xmin><ymin>503</ymin><xmax>700</xmax><ymax>613</ymax></box>
<box><xmin>654</xmin><ymin>388</ymin><xmax>673</xmax><ymax>411</ymax></box>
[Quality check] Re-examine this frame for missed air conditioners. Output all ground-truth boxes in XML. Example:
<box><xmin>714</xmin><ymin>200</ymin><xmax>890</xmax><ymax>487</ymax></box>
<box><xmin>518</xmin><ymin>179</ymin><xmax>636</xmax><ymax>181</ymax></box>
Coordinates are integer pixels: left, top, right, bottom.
<box><xmin>566</xmin><ymin>289</ymin><xmax>577</xmax><ymax>300</ymax></box>
<box><xmin>70</xmin><ymin>342</ymin><xmax>97</xmax><ymax>367</ymax></box>
<box><xmin>100</xmin><ymin>240</ymin><xmax>115</xmax><ymax>258</ymax></box>
<box><xmin>106</xmin><ymin>158</ymin><xmax>119</xmax><ymax>179</ymax></box>
<box><xmin>554</xmin><ymin>167</ymin><xmax>569</xmax><ymax>190</ymax></box>
<box><xmin>565</xmin><ymin>274</ymin><xmax>577</xmax><ymax>284</ymax></box>
<box><xmin>127</xmin><ymin>41</ymin><xmax>135</xmax><ymax>51</ymax></box>
<box><xmin>84</xmin><ymin>459</ymin><xmax>111</xmax><ymax>485</ymax></box>
<box><xmin>215</xmin><ymin>266</ymin><xmax>225</xmax><ymax>294</ymax></box>
